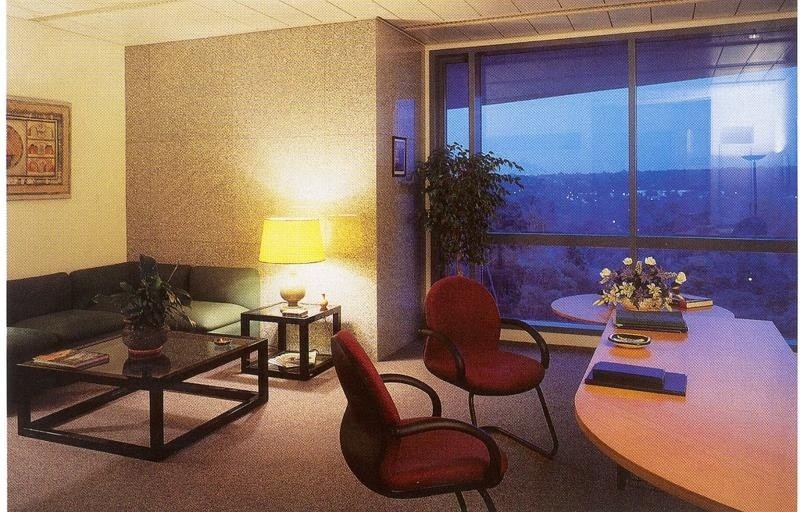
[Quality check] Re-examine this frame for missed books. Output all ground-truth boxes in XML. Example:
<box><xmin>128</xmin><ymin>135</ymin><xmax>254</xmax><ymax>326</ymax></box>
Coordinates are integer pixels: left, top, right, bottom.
<box><xmin>273</xmin><ymin>351</ymin><xmax>316</xmax><ymax>372</ymax></box>
<box><xmin>33</xmin><ymin>348</ymin><xmax>109</xmax><ymax>370</ymax></box>
<box><xmin>611</xmin><ymin>308</ymin><xmax>689</xmax><ymax>334</ymax></box>
<box><xmin>670</xmin><ymin>292</ymin><xmax>713</xmax><ymax>308</ymax></box>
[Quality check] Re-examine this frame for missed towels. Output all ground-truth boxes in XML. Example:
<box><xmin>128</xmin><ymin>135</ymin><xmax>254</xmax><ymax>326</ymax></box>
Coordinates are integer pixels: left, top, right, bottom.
<box><xmin>585</xmin><ymin>359</ymin><xmax>688</xmax><ymax>396</ymax></box>
<box><xmin>615</xmin><ymin>306</ymin><xmax>689</xmax><ymax>332</ymax></box>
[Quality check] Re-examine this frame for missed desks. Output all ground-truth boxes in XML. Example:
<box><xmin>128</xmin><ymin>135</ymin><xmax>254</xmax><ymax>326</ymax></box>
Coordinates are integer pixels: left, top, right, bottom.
<box><xmin>551</xmin><ymin>293</ymin><xmax>734</xmax><ymax>324</ymax></box>
<box><xmin>574</xmin><ymin>309</ymin><xmax>794</xmax><ymax>512</ymax></box>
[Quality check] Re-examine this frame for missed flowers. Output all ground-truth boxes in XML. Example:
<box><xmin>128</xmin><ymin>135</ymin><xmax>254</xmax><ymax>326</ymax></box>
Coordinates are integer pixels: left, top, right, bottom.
<box><xmin>593</xmin><ymin>256</ymin><xmax>685</xmax><ymax>314</ymax></box>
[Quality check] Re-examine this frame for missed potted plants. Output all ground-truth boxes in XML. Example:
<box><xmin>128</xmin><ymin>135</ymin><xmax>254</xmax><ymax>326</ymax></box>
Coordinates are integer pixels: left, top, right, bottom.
<box><xmin>90</xmin><ymin>253</ymin><xmax>197</xmax><ymax>362</ymax></box>
<box><xmin>398</xmin><ymin>140</ymin><xmax>525</xmax><ymax>279</ymax></box>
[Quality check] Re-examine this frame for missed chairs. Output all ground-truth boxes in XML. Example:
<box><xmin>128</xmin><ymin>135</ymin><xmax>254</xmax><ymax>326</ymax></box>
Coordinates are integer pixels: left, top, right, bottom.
<box><xmin>329</xmin><ymin>331</ymin><xmax>508</xmax><ymax>510</ymax></box>
<box><xmin>419</xmin><ymin>275</ymin><xmax>559</xmax><ymax>460</ymax></box>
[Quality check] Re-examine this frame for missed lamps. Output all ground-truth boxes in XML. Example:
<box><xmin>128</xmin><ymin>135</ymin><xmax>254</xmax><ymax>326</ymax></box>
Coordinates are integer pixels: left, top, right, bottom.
<box><xmin>257</xmin><ymin>217</ymin><xmax>324</xmax><ymax>314</ymax></box>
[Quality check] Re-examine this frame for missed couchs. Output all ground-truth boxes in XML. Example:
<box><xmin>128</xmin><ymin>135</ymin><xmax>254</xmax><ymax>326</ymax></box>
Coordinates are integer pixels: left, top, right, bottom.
<box><xmin>173</xmin><ymin>261</ymin><xmax>261</xmax><ymax>346</ymax></box>
<box><xmin>8</xmin><ymin>272</ymin><xmax>125</xmax><ymax>354</ymax></box>
<box><xmin>72</xmin><ymin>261</ymin><xmax>189</xmax><ymax>328</ymax></box>
<box><xmin>7</xmin><ymin>327</ymin><xmax>58</xmax><ymax>409</ymax></box>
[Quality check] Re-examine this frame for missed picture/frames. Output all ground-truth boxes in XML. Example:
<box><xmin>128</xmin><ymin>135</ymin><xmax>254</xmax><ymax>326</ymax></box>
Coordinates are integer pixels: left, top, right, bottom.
<box><xmin>7</xmin><ymin>93</ymin><xmax>71</xmax><ymax>201</ymax></box>
<box><xmin>392</xmin><ymin>136</ymin><xmax>407</xmax><ymax>176</ymax></box>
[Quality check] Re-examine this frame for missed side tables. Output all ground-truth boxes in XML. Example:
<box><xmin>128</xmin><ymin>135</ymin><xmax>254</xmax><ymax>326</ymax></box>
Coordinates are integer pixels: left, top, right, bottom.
<box><xmin>240</xmin><ymin>301</ymin><xmax>341</xmax><ymax>380</ymax></box>
